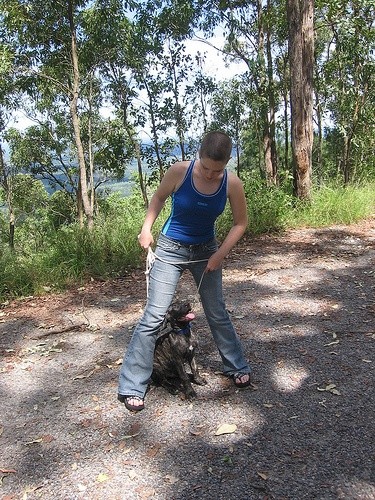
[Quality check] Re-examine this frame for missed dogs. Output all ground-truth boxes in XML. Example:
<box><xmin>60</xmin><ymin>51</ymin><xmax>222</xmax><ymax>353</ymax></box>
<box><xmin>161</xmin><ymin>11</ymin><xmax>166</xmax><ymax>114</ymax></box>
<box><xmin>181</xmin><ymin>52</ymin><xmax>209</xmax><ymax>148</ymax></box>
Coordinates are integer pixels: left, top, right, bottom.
<box><xmin>150</xmin><ymin>300</ymin><xmax>206</xmax><ymax>401</ymax></box>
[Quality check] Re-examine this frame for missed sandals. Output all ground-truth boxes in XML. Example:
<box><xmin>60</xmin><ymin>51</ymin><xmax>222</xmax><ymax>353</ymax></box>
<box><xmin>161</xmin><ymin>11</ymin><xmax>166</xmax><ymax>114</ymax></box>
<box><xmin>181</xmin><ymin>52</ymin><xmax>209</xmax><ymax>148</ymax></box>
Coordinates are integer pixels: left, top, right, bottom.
<box><xmin>233</xmin><ymin>372</ymin><xmax>250</xmax><ymax>388</ymax></box>
<box><xmin>118</xmin><ymin>395</ymin><xmax>144</xmax><ymax>411</ymax></box>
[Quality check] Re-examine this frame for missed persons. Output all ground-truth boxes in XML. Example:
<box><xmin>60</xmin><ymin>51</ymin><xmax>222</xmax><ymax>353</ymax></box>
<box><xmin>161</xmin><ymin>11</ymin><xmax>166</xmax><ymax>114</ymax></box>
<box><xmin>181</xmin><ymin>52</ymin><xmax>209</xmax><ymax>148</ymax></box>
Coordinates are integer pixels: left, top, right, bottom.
<box><xmin>118</xmin><ymin>131</ymin><xmax>252</xmax><ymax>410</ymax></box>
